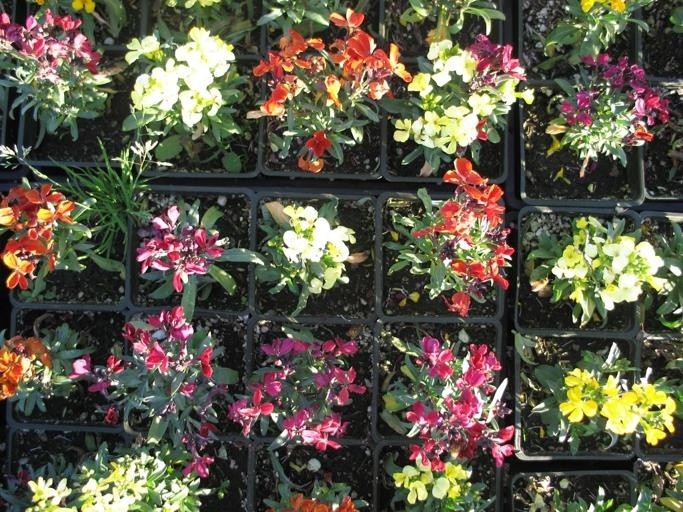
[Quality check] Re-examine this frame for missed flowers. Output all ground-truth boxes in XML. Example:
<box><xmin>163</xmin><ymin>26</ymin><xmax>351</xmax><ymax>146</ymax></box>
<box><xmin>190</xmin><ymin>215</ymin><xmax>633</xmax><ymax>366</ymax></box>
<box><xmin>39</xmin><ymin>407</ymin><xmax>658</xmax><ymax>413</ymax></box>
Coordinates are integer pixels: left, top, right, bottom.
<box><xmin>101</xmin><ymin>432</ymin><xmax>251</xmax><ymax>511</ymax></box>
<box><xmin>379</xmin><ymin>196</ymin><xmax>503</xmax><ymax>323</ymax></box>
<box><xmin>637</xmin><ymin>460</ymin><xmax>683</xmax><ymax>509</ymax></box>
<box><xmin>516</xmin><ymin>332</ymin><xmax>640</xmax><ymax>461</ymax></box>
<box><xmin>1</xmin><ymin>430</ymin><xmax>104</xmax><ymax>511</ymax></box>
<box><xmin>103</xmin><ymin>316</ymin><xmax>229</xmax><ymax>436</ymax></box>
<box><xmin>516</xmin><ymin>2</ymin><xmax>644</xmax><ymax>202</ymax></box>
<box><xmin>134</xmin><ymin>184</ymin><xmax>253</xmax><ymax>315</ymax></box>
<box><xmin>5</xmin><ymin>311</ymin><xmax>103</xmax><ymax>425</ymax></box>
<box><xmin>520</xmin><ymin>210</ymin><xmax>651</xmax><ymax>327</ymax></box>
<box><xmin>125</xmin><ymin>1</ymin><xmax>263</xmax><ymax>173</ymax></box>
<box><xmin>258</xmin><ymin>193</ymin><xmax>381</xmax><ymax>316</ymax></box>
<box><xmin>6</xmin><ymin>176</ymin><xmax>124</xmax><ymax>311</ymax></box>
<box><xmin>383</xmin><ymin>327</ymin><xmax>511</xmax><ymax>452</ymax></box>
<box><xmin>375</xmin><ymin>443</ymin><xmax>498</xmax><ymax>510</ymax></box>
<box><xmin>2</xmin><ymin>2</ymin><xmax>124</xmax><ymax>161</ymax></box>
<box><xmin>644</xmin><ymin>1</ymin><xmax>682</xmax><ymax>202</ymax></box>
<box><xmin>637</xmin><ymin>215</ymin><xmax>683</xmax><ymax>330</ymax></box>
<box><xmin>636</xmin><ymin>339</ymin><xmax>683</xmax><ymax>457</ymax></box>
<box><xmin>232</xmin><ymin>320</ymin><xmax>373</xmax><ymax>443</ymax></box>
<box><xmin>253</xmin><ymin>443</ymin><xmax>375</xmax><ymax>511</ymax></box>
<box><xmin>512</xmin><ymin>472</ymin><xmax>635</xmax><ymax>511</ymax></box>
<box><xmin>385</xmin><ymin>2</ymin><xmax>514</xmax><ymax>175</ymax></box>
<box><xmin>262</xmin><ymin>2</ymin><xmax>384</xmax><ymax>171</ymax></box>
